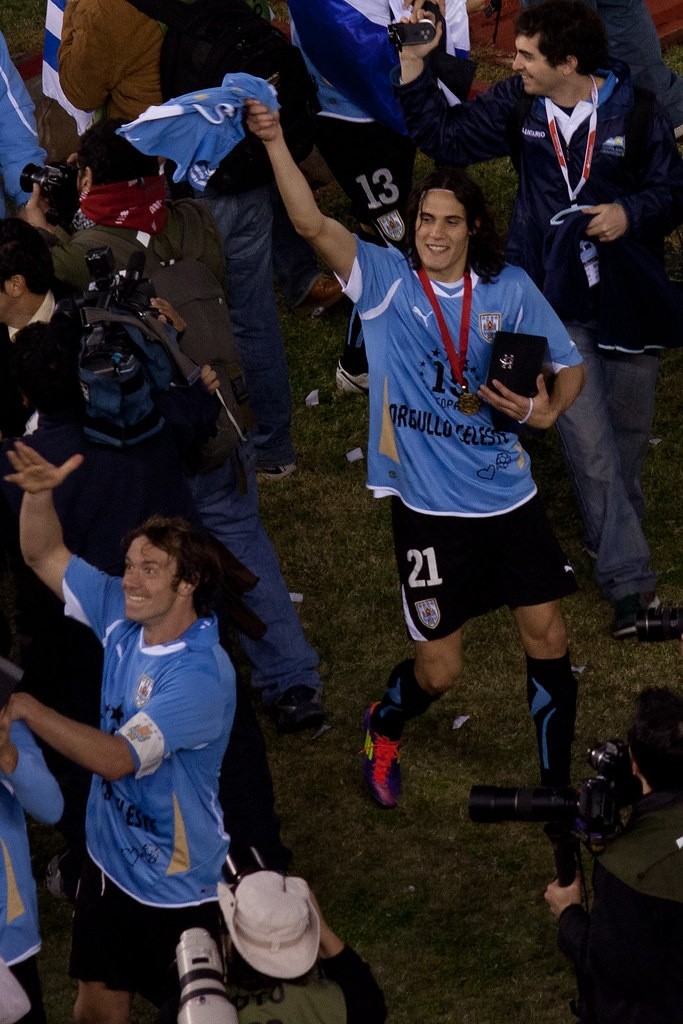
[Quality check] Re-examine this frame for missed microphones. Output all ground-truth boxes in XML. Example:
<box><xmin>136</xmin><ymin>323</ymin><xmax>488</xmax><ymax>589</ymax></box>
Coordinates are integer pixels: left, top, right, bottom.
<box><xmin>122</xmin><ymin>250</ymin><xmax>147</xmax><ymax>294</ymax></box>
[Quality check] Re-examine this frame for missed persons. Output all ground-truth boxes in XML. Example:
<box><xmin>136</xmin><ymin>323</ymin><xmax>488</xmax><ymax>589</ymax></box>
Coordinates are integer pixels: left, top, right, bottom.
<box><xmin>543</xmin><ymin>686</ymin><xmax>683</xmax><ymax>1024</ymax></box>
<box><xmin>1</xmin><ymin>443</ymin><xmax>237</xmax><ymax>1024</ymax></box>
<box><xmin>1</xmin><ymin>720</ymin><xmax>64</xmax><ymax>1024</ymax></box>
<box><xmin>0</xmin><ymin>0</ymin><xmax>683</xmax><ymax>806</ymax></box>
<box><xmin>217</xmin><ymin>870</ymin><xmax>387</xmax><ymax>1024</ymax></box>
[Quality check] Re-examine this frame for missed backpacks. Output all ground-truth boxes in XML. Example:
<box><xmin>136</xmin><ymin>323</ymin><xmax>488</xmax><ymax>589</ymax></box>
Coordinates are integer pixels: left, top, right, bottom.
<box><xmin>127</xmin><ymin>0</ymin><xmax>317</xmax><ymax>200</ymax></box>
<box><xmin>70</xmin><ymin>204</ymin><xmax>253</xmax><ymax>449</ymax></box>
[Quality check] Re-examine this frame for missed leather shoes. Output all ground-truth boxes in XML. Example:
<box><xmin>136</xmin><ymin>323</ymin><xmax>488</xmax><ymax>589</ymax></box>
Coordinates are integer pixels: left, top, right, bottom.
<box><xmin>293</xmin><ymin>275</ymin><xmax>344</xmax><ymax>318</ymax></box>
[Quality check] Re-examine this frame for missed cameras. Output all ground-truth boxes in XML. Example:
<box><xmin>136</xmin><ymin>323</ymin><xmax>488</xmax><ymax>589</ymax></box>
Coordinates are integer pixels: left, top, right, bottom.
<box><xmin>221</xmin><ymin>846</ymin><xmax>272</xmax><ymax>887</ymax></box>
<box><xmin>175</xmin><ymin>928</ymin><xmax>239</xmax><ymax>1024</ymax></box>
<box><xmin>19</xmin><ymin>162</ymin><xmax>81</xmax><ymax>214</ymax></box>
<box><xmin>387</xmin><ymin>19</ymin><xmax>437</xmax><ymax>53</ymax></box>
<box><xmin>470</xmin><ymin>737</ymin><xmax>636</xmax><ymax>837</ymax></box>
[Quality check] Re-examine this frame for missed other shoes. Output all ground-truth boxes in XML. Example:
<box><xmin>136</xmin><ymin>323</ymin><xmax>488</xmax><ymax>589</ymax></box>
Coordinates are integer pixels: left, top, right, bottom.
<box><xmin>255</xmin><ymin>463</ymin><xmax>297</xmax><ymax>479</ymax></box>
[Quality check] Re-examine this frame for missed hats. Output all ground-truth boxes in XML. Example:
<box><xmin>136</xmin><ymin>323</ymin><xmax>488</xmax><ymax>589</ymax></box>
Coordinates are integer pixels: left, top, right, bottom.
<box><xmin>218</xmin><ymin>819</ymin><xmax>320</xmax><ymax>979</ymax></box>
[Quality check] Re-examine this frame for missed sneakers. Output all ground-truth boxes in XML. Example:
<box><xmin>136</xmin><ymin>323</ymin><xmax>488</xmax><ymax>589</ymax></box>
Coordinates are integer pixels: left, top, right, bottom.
<box><xmin>270</xmin><ymin>685</ymin><xmax>325</xmax><ymax>733</ymax></box>
<box><xmin>613</xmin><ymin>594</ymin><xmax>683</xmax><ymax>641</ymax></box>
<box><xmin>362</xmin><ymin>702</ymin><xmax>403</xmax><ymax>806</ymax></box>
<box><xmin>303</xmin><ymin>359</ymin><xmax>370</xmax><ymax>408</ymax></box>
